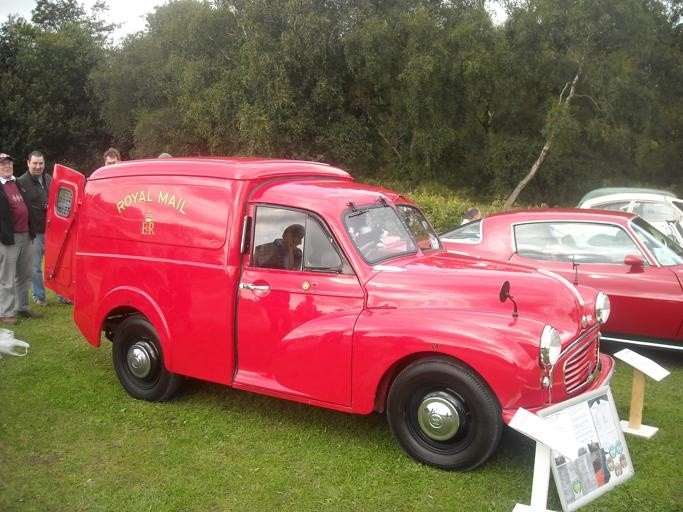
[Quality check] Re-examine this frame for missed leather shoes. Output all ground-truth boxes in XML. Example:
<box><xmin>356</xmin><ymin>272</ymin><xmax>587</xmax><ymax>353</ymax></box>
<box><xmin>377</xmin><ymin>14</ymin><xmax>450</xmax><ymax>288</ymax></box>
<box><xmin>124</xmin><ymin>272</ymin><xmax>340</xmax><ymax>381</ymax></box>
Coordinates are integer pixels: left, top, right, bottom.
<box><xmin>0</xmin><ymin>311</ymin><xmax>43</xmax><ymax>324</ymax></box>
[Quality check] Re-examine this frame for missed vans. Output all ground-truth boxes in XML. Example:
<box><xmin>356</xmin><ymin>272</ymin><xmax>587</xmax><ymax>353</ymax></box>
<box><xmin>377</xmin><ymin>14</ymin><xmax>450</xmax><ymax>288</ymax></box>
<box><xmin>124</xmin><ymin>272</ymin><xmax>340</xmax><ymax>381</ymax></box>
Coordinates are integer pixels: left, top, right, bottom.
<box><xmin>43</xmin><ymin>158</ymin><xmax>615</xmax><ymax>471</ymax></box>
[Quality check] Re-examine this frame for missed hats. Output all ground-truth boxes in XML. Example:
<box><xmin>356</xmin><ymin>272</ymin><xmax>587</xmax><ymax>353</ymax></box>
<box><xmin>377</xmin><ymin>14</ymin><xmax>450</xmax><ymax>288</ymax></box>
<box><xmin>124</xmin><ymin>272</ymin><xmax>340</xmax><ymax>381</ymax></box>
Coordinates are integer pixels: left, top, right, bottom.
<box><xmin>0</xmin><ymin>153</ymin><xmax>15</xmax><ymax>162</ymax></box>
<box><xmin>284</xmin><ymin>224</ymin><xmax>305</xmax><ymax>244</ymax></box>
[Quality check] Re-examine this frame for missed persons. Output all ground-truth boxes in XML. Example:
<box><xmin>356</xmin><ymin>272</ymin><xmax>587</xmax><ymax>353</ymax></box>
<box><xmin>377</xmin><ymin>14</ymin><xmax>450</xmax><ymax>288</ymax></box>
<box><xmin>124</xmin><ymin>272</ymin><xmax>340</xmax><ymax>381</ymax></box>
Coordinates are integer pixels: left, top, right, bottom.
<box><xmin>461</xmin><ymin>208</ymin><xmax>481</xmax><ymax>225</ymax></box>
<box><xmin>103</xmin><ymin>148</ymin><xmax>121</xmax><ymax>166</ymax></box>
<box><xmin>16</xmin><ymin>150</ymin><xmax>72</xmax><ymax>308</ymax></box>
<box><xmin>0</xmin><ymin>152</ymin><xmax>44</xmax><ymax>324</ymax></box>
<box><xmin>158</xmin><ymin>153</ymin><xmax>173</xmax><ymax>158</ymax></box>
<box><xmin>255</xmin><ymin>224</ymin><xmax>306</xmax><ymax>270</ymax></box>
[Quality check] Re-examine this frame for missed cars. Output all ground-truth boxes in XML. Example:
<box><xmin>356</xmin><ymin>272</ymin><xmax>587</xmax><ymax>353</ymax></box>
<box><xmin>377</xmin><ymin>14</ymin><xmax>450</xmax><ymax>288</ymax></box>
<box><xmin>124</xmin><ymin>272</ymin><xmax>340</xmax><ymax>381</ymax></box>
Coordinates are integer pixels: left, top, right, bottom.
<box><xmin>575</xmin><ymin>185</ymin><xmax>683</xmax><ymax>231</ymax></box>
<box><xmin>435</xmin><ymin>207</ymin><xmax>683</xmax><ymax>352</ymax></box>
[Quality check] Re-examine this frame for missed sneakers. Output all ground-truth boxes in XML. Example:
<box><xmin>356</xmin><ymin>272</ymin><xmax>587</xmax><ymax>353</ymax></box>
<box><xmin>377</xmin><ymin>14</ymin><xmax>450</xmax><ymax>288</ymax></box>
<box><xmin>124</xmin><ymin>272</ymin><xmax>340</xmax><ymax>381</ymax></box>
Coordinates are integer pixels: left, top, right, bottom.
<box><xmin>33</xmin><ymin>296</ymin><xmax>48</xmax><ymax>307</ymax></box>
<box><xmin>58</xmin><ymin>295</ymin><xmax>72</xmax><ymax>303</ymax></box>
